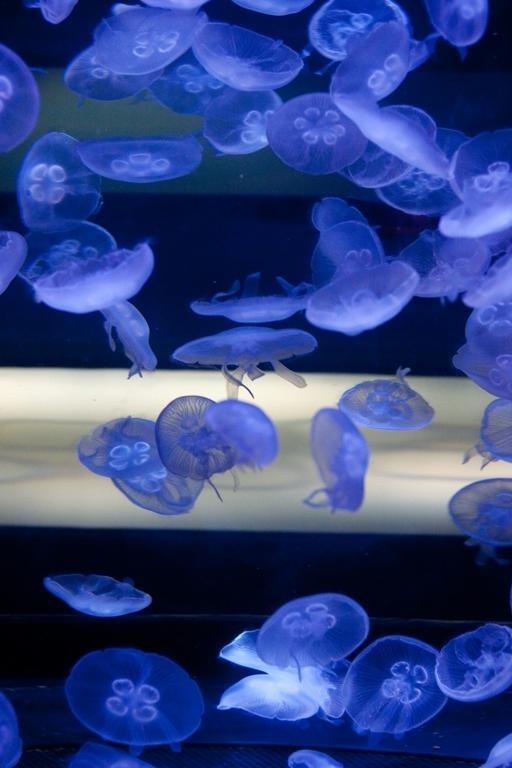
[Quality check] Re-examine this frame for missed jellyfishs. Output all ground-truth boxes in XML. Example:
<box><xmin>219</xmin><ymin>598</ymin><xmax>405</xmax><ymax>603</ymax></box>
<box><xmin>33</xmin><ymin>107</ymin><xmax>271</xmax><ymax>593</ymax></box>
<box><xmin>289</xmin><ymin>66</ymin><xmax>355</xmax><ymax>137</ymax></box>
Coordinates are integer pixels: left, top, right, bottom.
<box><xmin>0</xmin><ymin>1</ymin><xmax>512</xmax><ymax>768</ymax></box>
